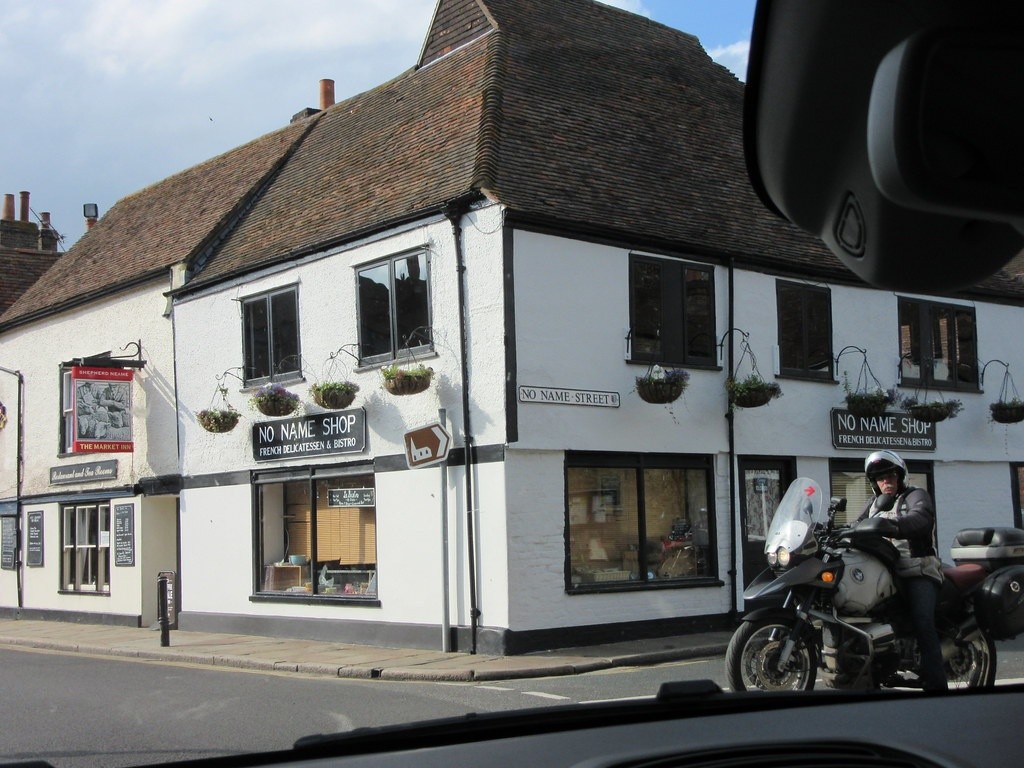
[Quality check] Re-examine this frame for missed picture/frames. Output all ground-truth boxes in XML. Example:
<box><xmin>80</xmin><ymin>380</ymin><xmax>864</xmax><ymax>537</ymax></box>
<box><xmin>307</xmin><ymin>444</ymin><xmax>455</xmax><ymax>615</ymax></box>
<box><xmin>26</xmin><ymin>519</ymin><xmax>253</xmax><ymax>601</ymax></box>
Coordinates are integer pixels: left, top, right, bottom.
<box><xmin>27</xmin><ymin>510</ymin><xmax>43</xmax><ymax>567</ymax></box>
<box><xmin>114</xmin><ymin>502</ymin><xmax>136</xmax><ymax>568</ymax></box>
<box><xmin>1</xmin><ymin>517</ymin><xmax>17</xmax><ymax>570</ymax></box>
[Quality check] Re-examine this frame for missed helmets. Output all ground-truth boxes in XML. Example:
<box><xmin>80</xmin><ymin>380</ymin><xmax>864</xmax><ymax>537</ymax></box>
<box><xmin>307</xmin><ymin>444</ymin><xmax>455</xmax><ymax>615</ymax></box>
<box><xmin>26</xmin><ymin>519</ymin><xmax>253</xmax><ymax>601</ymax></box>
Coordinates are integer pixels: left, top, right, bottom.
<box><xmin>865</xmin><ymin>449</ymin><xmax>909</xmax><ymax>496</ymax></box>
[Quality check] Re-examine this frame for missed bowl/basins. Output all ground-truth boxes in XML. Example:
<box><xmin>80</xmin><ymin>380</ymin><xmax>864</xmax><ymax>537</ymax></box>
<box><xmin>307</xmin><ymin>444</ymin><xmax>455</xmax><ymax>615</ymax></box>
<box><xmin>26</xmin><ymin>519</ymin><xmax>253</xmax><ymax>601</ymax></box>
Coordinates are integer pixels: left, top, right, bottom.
<box><xmin>290</xmin><ymin>555</ymin><xmax>306</xmax><ymax>565</ymax></box>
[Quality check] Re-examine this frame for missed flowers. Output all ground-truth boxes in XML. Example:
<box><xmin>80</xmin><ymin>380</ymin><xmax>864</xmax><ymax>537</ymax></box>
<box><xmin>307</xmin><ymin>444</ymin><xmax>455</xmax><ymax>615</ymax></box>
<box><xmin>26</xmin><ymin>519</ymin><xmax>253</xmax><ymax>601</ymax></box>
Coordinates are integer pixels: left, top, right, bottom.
<box><xmin>195</xmin><ymin>407</ymin><xmax>243</xmax><ymax>433</ymax></box>
<box><xmin>988</xmin><ymin>397</ymin><xmax>1024</xmax><ymax>410</ymax></box>
<box><xmin>840</xmin><ymin>370</ymin><xmax>904</xmax><ymax>409</ymax></box>
<box><xmin>726</xmin><ymin>374</ymin><xmax>784</xmax><ymax>405</ymax></box>
<box><xmin>628</xmin><ymin>367</ymin><xmax>690</xmax><ymax>394</ymax></box>
<box><xmin>248</xmin><ymin>382</ymin><xmax>301</xmax><ymax>416</ymax></box>
<box><xmin>899</xmin><ymin>396</ymin><xmax>964</xmax><ymax>419</ymax></box>
<box><xmin>379</xmin><ymin>364</ymin><xmax>434</xmax><ymax>390</ymax></box>
<box><xmin>306</xmin><ymin>380</ymin><xmax>360</xmax><ymax>406</ymax></box>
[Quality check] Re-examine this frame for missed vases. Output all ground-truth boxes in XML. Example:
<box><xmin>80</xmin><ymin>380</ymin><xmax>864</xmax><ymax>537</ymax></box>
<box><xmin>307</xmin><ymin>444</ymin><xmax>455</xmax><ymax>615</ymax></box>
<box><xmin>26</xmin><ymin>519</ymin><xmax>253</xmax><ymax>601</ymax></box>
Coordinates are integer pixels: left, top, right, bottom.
<box><xmin>912</xmin><ymin>408</ymin><xmax>951</xmax><ymax>423</ymax></box>
<box><xmin>383</xmin><ymin>375</ymin><xmax>430</xmax><ymax>396</ymax></box>
<box><xmin>991</xmin><ymin>409</ymin><xmax>1024</xmax><ymax>423</ymax></box>
<box><xmin>258</xmin><ymin>401</ymin><xmax>294</xmax><ymax>416</ymax></box>
<box><xmin>848</xmin><ymin>402</ymin><xmax>886</xmax><ymax>417</ymax></box>
<box><xmin>204</xmin><ymin>419</ymin><xmax>239</xmax><ymax>433</ymax></box>
<box><xmin>314</xmin><ymin>393</ymin><xmax>354</xmax><ymax>409</ymax></box>
<box><xmin>735</xmin><ymin>392</ymin><xmax>773</xmax><ymax>408</ymax></box>
<box><xmin>637</xmin><ymin>385</ymin><xmax>682</xmax><ymax>404</ymax></box>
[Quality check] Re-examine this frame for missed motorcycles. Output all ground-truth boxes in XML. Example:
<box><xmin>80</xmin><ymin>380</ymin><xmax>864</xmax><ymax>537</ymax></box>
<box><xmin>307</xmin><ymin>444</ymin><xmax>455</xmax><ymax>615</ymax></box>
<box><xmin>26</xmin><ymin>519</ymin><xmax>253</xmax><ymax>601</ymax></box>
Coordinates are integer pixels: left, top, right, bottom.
<box><xmin>726</xmin><ymin>476</ymin><xmax>1024</xmax><ymax>700</ymax></box>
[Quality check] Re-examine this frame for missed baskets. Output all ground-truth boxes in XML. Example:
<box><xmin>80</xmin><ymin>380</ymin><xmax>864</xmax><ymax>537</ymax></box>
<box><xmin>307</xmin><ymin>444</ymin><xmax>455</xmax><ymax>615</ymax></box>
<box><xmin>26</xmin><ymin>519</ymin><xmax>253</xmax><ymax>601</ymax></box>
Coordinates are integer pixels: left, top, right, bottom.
<box><xmin>847</xmin><ymin>359</ymin><xmax>886</xmax><ymax>417</ymax></box>
<box><xmin>199</xmin><ymin>382</ymin><xmax>238</xmax><ymax>433</ymax></box>
<box><xmin>253</xmin><ymin>369</ymin><xmax>299</xmax><ymax>416</ymax></box>
<box><xmin>385</xmin><ymin>342</ymin><xmax>431</xmax><ymax>395</ymax></box>
<box><xmin>635</xmin><ymin>338</ymin><xmax>682</xmax><ymax>404</ymax></box>
<box><xmin>312</xmin><ymin>359</ymin><xmax>357</xmax><ymax>409</ymax></box>
<box><xmin>910</xmin><ymin>365</ymin><xmax>951</xmax><ymax>423</ymax></box>
<box><xmin>992</xmin><ymin>371</ymin><xmax>1024</xmax><ymax>423</ymax></box>
<box><xmin>732</xmin><ymin>343</ymin><xmax>771</xmax><ymax>407</ymax></box>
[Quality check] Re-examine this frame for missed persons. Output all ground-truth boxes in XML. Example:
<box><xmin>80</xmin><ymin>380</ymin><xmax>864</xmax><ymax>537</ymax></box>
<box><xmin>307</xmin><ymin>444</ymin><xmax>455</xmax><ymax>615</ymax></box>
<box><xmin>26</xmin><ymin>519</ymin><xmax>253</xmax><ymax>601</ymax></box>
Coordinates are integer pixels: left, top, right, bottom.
<box><xmin>811</xmin><ymin>449</ymin><xmax>952</xmax><ymax>694</ymax></box>
